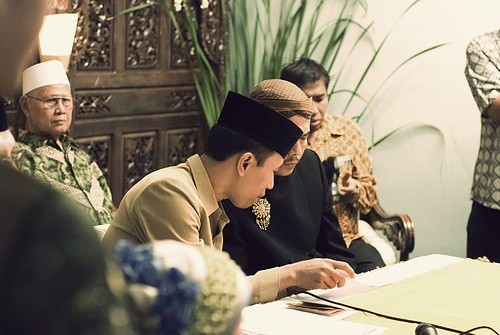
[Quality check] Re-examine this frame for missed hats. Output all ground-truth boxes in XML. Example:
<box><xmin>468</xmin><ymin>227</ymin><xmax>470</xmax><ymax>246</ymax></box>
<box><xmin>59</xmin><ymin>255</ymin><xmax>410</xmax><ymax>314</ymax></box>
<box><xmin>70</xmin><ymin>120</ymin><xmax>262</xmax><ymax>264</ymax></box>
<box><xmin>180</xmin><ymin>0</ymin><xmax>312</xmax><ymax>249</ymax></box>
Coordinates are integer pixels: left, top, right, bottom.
<box><xmin>115</xmin><ymin>239</ymin><xmax>246</xmax><ymax>335</ymax></box>
<box><xmin>248</xmin><ymin>79</ymin><xmax>313</xmax><ymax>120</ymax></box>
<box><xmin>218</xmin><ymin>91</ymin><xmax>303</xmax><ymax>159</ymax></box>
<box><xmin>22</xmin><ymin>60</ymin><xmax>71</xmax><ymax>96</ymax></box>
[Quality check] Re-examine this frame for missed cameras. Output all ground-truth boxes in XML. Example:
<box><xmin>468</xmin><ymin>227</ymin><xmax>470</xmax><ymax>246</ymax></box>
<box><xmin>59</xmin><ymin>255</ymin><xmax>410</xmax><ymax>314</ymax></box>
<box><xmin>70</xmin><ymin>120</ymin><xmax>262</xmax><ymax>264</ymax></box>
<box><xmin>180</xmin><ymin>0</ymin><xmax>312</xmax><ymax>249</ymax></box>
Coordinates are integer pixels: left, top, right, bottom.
<box><xmin>321</xmin><ymin>153</ymin><xmax>344</xmax><ymax>204</ymax></box>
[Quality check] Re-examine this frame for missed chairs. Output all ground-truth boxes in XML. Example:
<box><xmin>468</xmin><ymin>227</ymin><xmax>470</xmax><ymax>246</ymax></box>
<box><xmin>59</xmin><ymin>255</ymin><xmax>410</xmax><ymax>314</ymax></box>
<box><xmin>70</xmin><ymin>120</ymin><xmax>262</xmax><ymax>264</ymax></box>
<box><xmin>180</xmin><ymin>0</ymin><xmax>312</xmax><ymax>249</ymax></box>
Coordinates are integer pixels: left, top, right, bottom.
<box><xmin>360</xmin><ymin>182</ymin><xmax>416</xmax><ymax>263</ymax></box>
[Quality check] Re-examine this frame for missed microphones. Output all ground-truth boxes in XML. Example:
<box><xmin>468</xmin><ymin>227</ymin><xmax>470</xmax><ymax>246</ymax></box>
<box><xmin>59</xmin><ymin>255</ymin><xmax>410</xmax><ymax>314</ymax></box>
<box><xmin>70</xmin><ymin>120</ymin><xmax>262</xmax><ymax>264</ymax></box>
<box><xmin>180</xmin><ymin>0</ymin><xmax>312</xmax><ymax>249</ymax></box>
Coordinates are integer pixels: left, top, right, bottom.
<box><xmin>415</xmin><ymin>323</ymin><xmax>437</xmax><ymax>335</ymax></box>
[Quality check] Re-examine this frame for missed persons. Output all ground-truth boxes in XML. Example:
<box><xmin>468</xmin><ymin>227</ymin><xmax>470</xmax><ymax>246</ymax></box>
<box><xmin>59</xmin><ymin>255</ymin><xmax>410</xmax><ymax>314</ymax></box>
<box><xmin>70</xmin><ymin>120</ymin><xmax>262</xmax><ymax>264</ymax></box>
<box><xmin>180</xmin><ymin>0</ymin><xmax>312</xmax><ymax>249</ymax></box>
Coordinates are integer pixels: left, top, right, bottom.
<box><xmin>101</xmin><ymin>91</ymin><xmax>357</xmax><ymax>306</ymax></box>
<box><xmin>0</xmin><ymin>59</ymin><xmax>119</xmax><ymax>227</ymax></box>
<box><xmin>0</xmin><ymin>0</ymin><xmax>252</xmax><ymax>335</ymax></box>
<box><xmin>221</xmin><ymin>79</ymin><xmax>357</xmax><ymax>298</ymax></box>
<box><xmin>464</xmin><ymin>29</ymin><xmax>500</xmax><ymax>264</ymax></box>
<box><xmin>281</xmin><ymin>58</ymin><xmax>386</xmax><ymax>274</ymax></box>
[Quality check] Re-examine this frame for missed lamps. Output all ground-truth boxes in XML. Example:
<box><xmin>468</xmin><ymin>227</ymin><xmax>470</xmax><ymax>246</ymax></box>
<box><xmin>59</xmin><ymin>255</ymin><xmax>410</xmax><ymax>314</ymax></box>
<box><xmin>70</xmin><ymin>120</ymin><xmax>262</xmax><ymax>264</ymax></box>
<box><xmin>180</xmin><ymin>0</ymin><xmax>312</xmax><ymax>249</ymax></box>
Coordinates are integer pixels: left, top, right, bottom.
<box><xmin>38</xmin><ymin>12</ymin><xmax>80</xmax><ymax>73</ymax></box>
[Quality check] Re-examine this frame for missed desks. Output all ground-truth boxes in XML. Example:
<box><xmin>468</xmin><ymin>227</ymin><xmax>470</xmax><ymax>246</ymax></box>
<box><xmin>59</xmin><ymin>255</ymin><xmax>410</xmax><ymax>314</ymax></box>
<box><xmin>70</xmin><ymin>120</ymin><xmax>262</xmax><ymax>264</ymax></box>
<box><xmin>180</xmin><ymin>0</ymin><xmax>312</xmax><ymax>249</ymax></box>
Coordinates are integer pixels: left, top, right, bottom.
<box><xmin>232</xmin><ymin>254</ymin><xmax>466</xmax><ymax>335</ymax></box>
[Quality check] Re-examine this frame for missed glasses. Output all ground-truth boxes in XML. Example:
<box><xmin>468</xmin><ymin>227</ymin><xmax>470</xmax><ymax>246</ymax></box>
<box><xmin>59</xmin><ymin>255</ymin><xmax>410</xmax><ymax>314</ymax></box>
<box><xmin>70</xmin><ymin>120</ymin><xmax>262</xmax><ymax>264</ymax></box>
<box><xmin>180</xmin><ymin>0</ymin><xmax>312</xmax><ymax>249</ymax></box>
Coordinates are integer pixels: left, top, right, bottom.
<box><xmin>26</xmin><ymin>95</ymin><xmax>73</xmax><ymax>109</ymax></box>
